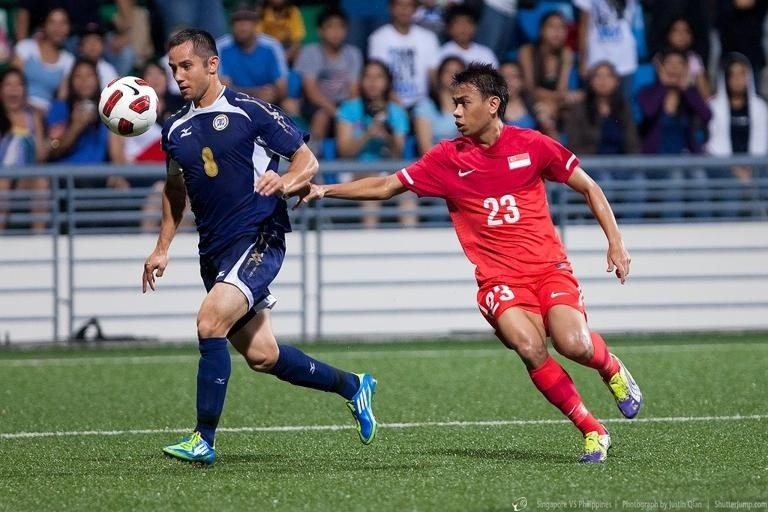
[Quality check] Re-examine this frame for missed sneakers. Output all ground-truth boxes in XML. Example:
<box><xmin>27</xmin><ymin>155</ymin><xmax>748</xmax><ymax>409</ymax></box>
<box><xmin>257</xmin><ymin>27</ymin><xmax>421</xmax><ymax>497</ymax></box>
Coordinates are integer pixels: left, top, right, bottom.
<box><xmin>579</xmin><ymin>422</ymin><xmax>613</xmax><ymax>465</ymax></box>
<box><xmin>600</xmin><ymin>352</ymin><xmax>643</xmax><ymax>419</ymax></box>
<box><xmin>163</xmin><ymin>432</ymin><xmax>217</xmax><ymax>465</ymax></box>
<box><xmin>345</xmin><ymin>371</ymin><xmax>378</xmax><ymax>444</ymax></box>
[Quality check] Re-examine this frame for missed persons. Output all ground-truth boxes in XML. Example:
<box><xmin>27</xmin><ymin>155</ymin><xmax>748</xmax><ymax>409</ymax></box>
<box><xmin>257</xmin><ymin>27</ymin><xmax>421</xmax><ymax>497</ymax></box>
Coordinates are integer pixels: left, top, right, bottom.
<box><xmin>140</xmin><ymin>25</ymin><xmax>380</xmax><ymax>467</ymax></box>
<box><xmin>0</xmin><ymin>0</ymin><xmax>768</xmax><ymax>232</ymax></box>
<box><xmin>282</xmin><ymin>59</ymin><xmax>644</xmax><ymax>466</ymax></box>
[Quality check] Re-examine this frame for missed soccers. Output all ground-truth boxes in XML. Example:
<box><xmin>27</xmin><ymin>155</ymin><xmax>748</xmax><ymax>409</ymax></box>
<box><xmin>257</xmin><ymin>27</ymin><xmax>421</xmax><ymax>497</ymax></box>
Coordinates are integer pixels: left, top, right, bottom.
<box><xmin>99</xmin><ymin>76</ymin><xmax>160</xmax><ymax>137</ymax></box>
